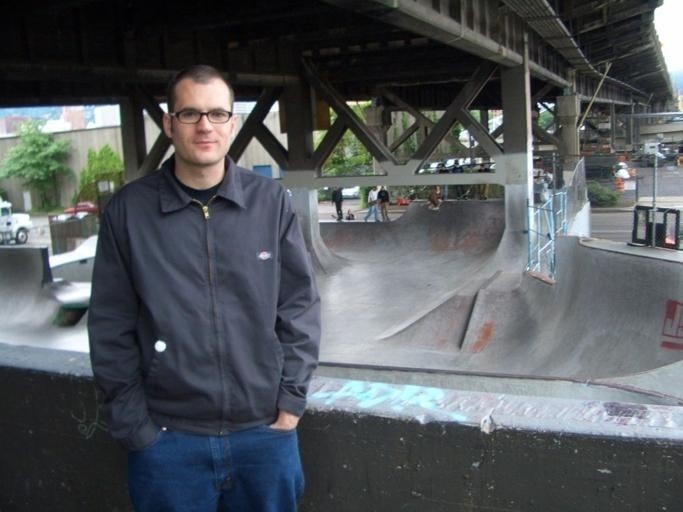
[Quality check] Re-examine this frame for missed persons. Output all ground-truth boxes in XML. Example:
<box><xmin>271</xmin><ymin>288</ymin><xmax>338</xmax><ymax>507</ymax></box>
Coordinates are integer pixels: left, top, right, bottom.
<box><xmin>438</xmin><ymin>163</ymin><xmax>449</xmax><ymax>199</ymax></box>
<box><xmin>90</xmin><ymin>60</ymin><xmax>320</xmax><ymax>511</ymax></box>
<box><xmin>364</xmin><ymin>187</ymin><xmax>378</xmax><ymax>221</ymax></box>
<box><xmin>377</xmin><ymin>186</ymin><xmax>390</xmax><ymax>220</ymax></box>
<box><xmin>476</xmin><ymin>163</ymin><xmax>491</xmax><ymax>199</ymax></box>
<box><xmin>330</xmin><ymin>187</ymin><xmax>343</xmax><ymax>220</ymax></box>
<box><xmin>450</xmin><ymin>161</ymin><xmax>464</xmax><ymax>199</ymax></box>
<box><xmin>345</xmin><ymin>208</ymin><xmax>355</xmax><ymax>220</ymax></box>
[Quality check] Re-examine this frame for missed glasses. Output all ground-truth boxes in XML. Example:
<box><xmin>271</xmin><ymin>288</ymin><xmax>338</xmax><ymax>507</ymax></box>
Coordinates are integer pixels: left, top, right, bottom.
<box><xmin>170</xmin><ymin>108</ymin><xmax>232</xmax><ymax>124</ymax></box>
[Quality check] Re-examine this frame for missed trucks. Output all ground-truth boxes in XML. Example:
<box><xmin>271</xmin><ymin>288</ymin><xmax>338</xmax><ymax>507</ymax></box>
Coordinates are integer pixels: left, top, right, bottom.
<box><xmin>0</xmin><ymin>200</ymin><xmax>34</xmax><ymax>244</ymax></box>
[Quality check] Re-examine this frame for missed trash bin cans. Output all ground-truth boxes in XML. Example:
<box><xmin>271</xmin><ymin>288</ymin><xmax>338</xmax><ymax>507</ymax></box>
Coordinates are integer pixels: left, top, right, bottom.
<box><xmin>48</xmin><ymin>211</ymin><xmax>97</xmax><ymax>256</ymax></box>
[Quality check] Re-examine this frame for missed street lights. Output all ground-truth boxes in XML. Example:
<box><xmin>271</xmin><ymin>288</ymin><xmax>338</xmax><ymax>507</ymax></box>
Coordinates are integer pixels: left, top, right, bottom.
<box><xmin>656</xmin><ymin>129</ymin><xmax>665</xmax><ymax>153</ymax></box>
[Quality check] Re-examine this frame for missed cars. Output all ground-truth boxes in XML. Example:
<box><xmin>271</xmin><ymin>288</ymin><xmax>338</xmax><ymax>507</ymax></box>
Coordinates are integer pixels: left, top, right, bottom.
<box><xmin>63</xmin><ymin>201</ymin><xmax>98</xmax><ymax>214</ymax></box>
<box><xmin>340</xmin><ymin>157</ymin><xmax>479</xmax><ymax>199</ymax></box>
